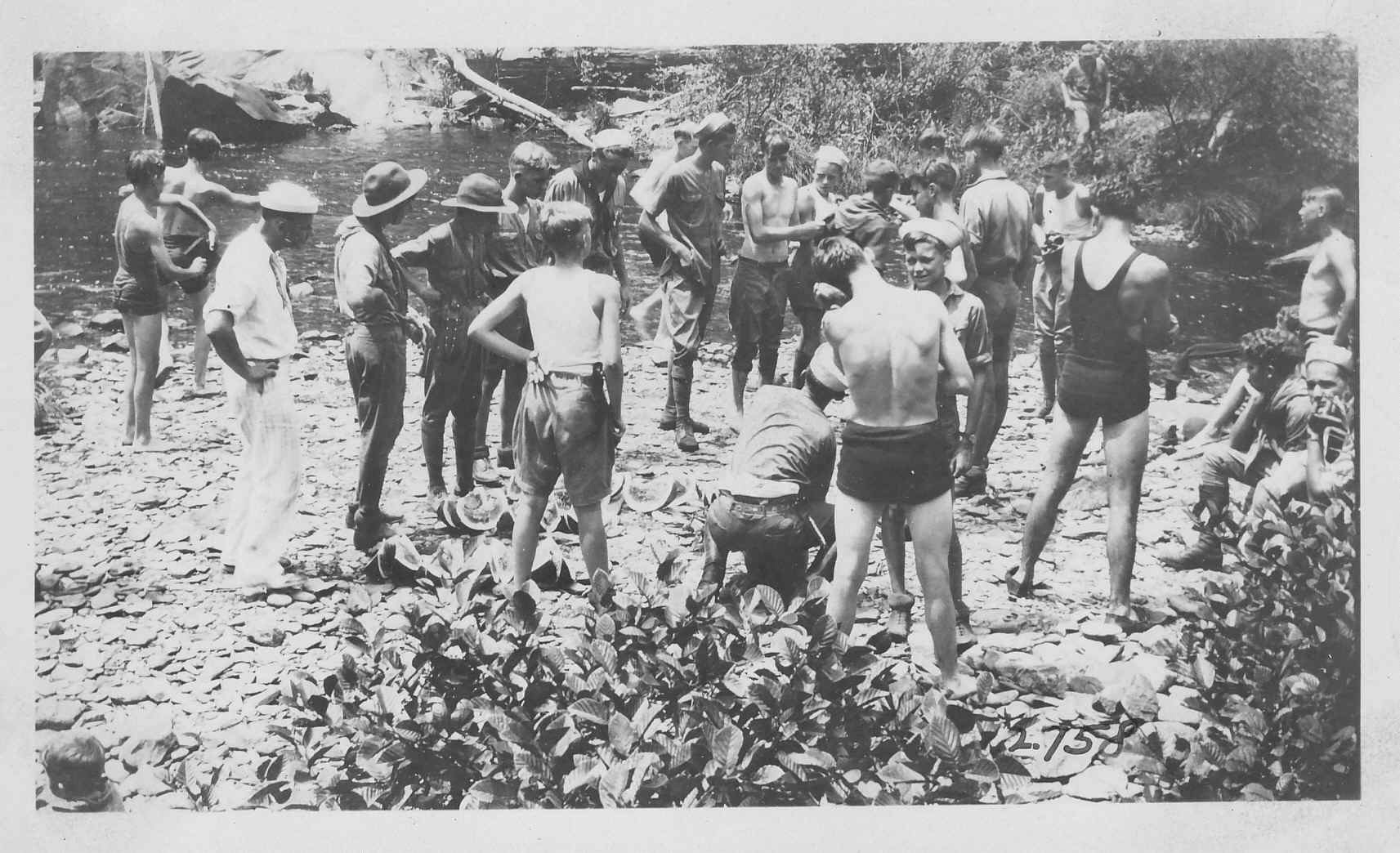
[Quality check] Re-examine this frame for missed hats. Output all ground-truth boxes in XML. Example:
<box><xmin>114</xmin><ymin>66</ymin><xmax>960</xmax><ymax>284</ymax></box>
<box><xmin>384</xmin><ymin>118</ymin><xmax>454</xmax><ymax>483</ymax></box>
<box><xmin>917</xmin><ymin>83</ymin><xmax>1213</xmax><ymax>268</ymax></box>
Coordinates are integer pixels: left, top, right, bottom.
<box><xmin>809</xmin><ymin>341</ymin><xmax>850</xmax><ymax>392</ymax></box>
<box><xmin>1306</xmin><ymin>343</ymin><xmax>1354</xmax><ymax>372</ymax></box>
<box><xmin>259</xmin><ymin>181</ymin><xmax>319</xmax><ymax>213</ymax></box>
<box><xmin>353</xmin><ymin>161</ymin><xmax>429</xmax><ymax>217</ymax></box>
<box><xmin>693</xmin><ymin>110</ymin><xmax>727</xmax><ymax>141</ymax></box>
<box><xmin>593</xmin><ymin>129</ymin><xmax>633</xmax><ymax>149</ymax></box>
<box><xmin>442</xmin><ymin>172</ymin><xmax>519</xmax><ymax>213</ymax></box>
<box><xmin>816</xmin><ymin>146</ymin><xmax>849</xmax><ymax>170</ymax></box>
<box><xmin>898</xmin><ymin>217</ymin><xmax>963</xmax><ymax>249</ymax></box>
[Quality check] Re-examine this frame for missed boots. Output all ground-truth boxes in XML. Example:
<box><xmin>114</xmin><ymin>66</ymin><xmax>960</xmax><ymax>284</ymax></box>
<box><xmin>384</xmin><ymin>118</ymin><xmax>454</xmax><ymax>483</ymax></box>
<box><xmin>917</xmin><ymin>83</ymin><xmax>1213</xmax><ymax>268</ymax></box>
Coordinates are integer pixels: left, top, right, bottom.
<box><xmin>887</xmin><ymin>592</ymin><xmax>914</xmax><ymax>642</ymax></box>
<box><xmin>952</xmin><ymin>606</ymin><xmax>978</xmax><ymax>655</ymax></box>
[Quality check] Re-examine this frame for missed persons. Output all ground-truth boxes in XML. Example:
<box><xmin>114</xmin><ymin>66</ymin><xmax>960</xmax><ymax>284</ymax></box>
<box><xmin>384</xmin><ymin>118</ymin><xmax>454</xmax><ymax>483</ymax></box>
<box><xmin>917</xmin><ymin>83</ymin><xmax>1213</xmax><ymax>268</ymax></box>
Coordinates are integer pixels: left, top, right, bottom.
<box><xmin>1160</xmin><ymin>186</ymin><xmax>1360</xmax><ymax>571</ymax></box>
<box><xmin>110</xmin><ymin>112</ymin><xmax>1180</xmax><ymax>698</ymax></box>
<box><xmin>696</xmin><ymin>343</ymin><xmax>852</xmax><ymax>598</ymax></box>
<box><xmin>468</xmin><ymin>200</ymin><xmax>625</xmax><ymax>585</ymax></box>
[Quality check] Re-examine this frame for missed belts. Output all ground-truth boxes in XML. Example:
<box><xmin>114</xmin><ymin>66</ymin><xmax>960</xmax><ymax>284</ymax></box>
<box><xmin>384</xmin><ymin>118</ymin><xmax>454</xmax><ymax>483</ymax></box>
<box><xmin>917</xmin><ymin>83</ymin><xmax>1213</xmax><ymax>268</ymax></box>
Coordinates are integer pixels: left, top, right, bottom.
<box><xmin>720</xmin><ymin>495</ymin><xmax>803</xmax><ymax>514</ymax></box>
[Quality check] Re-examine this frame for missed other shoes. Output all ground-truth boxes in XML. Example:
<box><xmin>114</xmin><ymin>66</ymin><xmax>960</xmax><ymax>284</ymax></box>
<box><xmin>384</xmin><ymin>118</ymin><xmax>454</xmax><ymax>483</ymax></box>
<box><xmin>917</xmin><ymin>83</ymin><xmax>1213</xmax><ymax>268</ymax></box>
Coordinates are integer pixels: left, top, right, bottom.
<box><xmin>1104</xmin><ymin>605</ymin><xmax>1168</xmax><ymax>635</ymax></box>
<box><xmin>223</xmin><ymin>556</ymin><xmax>291</xmax><ymax>572</ymax></box>
<box><xmin>354</xmin><ymin>529</ymin><xmax>396</xmax><ymax>551</ymax></box>
<box><xmin>953</xmin><ymin>476</ymin><xmax>987</xmax><ymax>497</ymax></box>
<box><xmin>346</xmin><ymin>503</ymin><xmax>405</xmax><ymax>529</ymax></box>
<box><xmin>1158</xmin><ymin>545</ymin><xmax>1223</xmax><ymax>570</ymax></box>
<box><xmin>686</xmin><ymin>589</ymin><xmax>708</xmax><ymax>616</ymax></box>
<box><xmin>1007</xmin><ymin>566</ymin><xmax>1033</xmax><ymax>596</ymax></box>
<box><xmin>426</xmin><ymin>488</ymin><xmax>450</xmax><ymax>512</ymax></box>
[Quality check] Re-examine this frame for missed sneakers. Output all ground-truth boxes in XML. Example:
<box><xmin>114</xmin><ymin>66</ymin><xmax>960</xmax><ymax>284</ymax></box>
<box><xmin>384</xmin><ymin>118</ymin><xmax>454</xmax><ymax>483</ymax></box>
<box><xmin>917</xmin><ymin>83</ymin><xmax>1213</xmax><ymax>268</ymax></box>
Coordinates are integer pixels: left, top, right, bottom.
<box><xmin>674</xmin><ymin>417</ymin><xmax>699</xmax><ymax>450</ymax></box>
<box><xmin>474</xmin><ymin>458</ymin><xmax>502</xmax><ymax>481</ymax></box>
<box><xmin>661</xmin><ymin>417</ymin><xmax>710</xmax><ymax>433</ymax></box>
